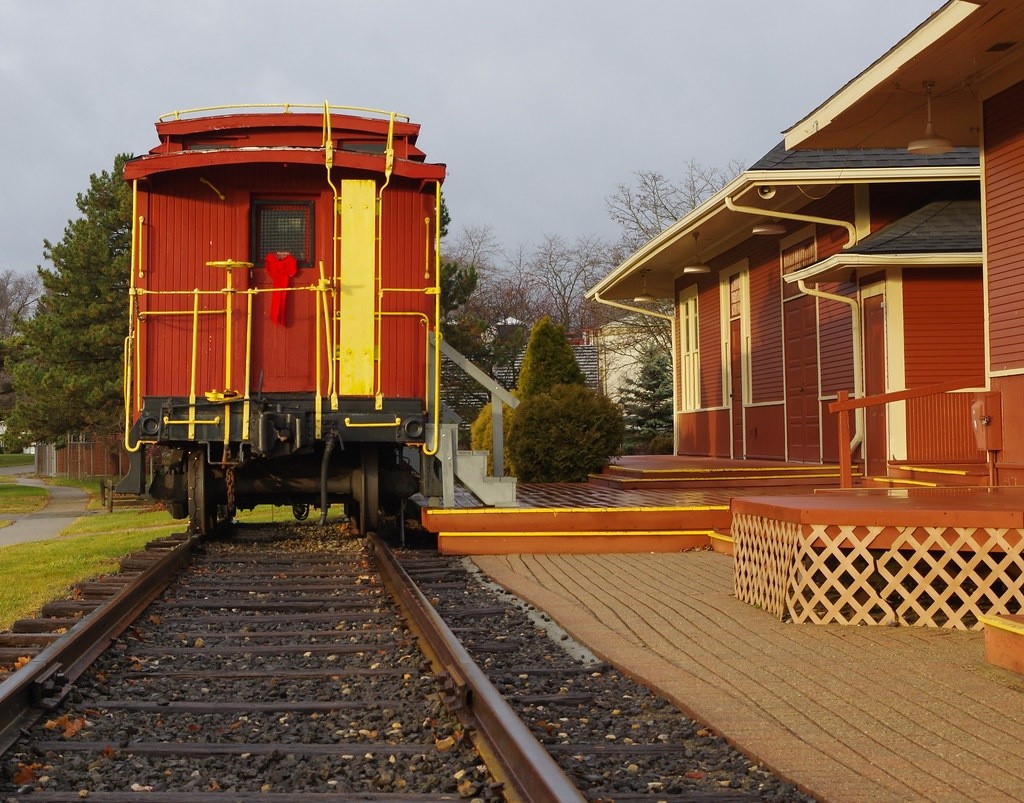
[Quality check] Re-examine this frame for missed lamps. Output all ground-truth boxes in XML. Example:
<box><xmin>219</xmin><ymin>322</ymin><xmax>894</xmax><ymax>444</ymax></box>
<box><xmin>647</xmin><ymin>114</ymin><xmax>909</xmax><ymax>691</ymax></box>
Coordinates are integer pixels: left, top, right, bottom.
<box><xmin>634</xmin><ymin>270</ymin><xmax>656</xmax><ymax>303</ymax></box>
<box><xmin>752</xmin><ymin>205</ymin><xmax>787</xmax><ymax>235</ymax></box>
<box><xmin>906</xmin><ymin>79</ymin><xmax>955</xmax><ymax>154</ymax></box>
<box><xmin>683</xmin><ymin>233</ymin><xmax>711</xmax><ymax>274</ymax></box>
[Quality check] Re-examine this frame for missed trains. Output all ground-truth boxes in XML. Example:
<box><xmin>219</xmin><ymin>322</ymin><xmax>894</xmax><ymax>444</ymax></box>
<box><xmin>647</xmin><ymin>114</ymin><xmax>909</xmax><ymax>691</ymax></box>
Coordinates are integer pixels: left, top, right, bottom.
<box><xmin>118</xmin><ymin>101</ymin><xmax>454</xmax><ymax>535</ymax></box>
